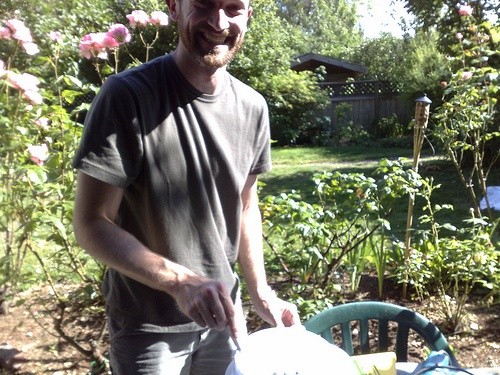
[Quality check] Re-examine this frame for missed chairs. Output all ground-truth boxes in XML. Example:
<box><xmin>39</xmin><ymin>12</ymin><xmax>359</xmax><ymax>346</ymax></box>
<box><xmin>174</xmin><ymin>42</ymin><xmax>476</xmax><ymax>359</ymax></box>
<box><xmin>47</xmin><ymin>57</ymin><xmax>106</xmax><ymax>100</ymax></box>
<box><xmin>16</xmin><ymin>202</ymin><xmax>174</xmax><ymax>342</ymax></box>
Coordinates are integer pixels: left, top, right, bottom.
<box><xmin>301</xmin><ymin>300</ymin><xmax>459</xmax><ymax>369</ymax></box>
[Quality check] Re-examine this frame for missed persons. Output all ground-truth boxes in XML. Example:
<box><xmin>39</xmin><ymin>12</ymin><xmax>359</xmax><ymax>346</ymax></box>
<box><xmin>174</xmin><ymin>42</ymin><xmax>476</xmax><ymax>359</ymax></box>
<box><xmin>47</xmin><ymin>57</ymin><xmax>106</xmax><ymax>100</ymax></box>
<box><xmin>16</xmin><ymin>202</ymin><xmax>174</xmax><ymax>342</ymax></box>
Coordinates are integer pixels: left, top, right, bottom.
<box><xmin>73</xmin><ymin>0</ymin><xmax>303</xmax><ymax>374</ymax></box>
